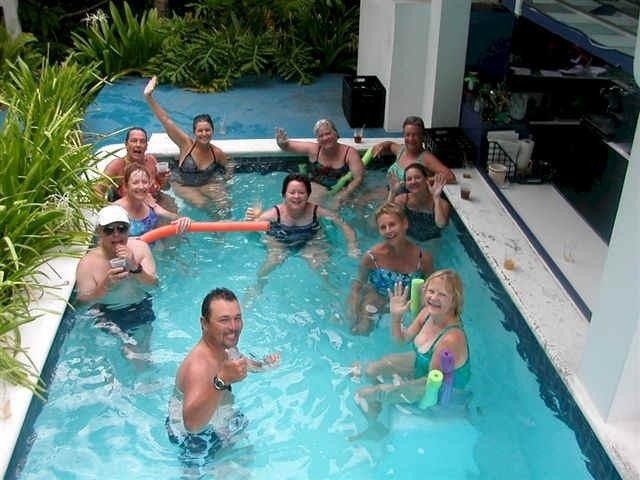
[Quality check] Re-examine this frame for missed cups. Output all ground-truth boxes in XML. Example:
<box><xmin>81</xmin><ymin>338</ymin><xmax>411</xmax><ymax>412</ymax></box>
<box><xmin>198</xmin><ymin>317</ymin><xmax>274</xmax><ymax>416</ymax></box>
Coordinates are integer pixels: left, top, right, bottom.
<box><xmin>156</xmin><ymin>162</ymin><xmax>168</xmax><ymax>186</ymax></box>
<box><xmin>251</xmin><ymin>200</ymin><xmax>263</xmax><ymax>217</ymax></box>
<box><xmin>504</xmin><ymin>248</ymin><xmax>514</xmax><ymax>269</ymax></box>
<box><xmin>111</xmin><ymin>258</ymin><xmax>127</xmax><ymax>275</ymax></box>
<box><xmin>460</xmin><ymin>182</ymin><xmax>472</xmax><ymax>200</ymax></box>
<box><xmin>353</xmin><ymin>128</ymin><xmax>363</xmax><ymax>142</ymax></box>
<box><xmin>562</xmin><ymin>240</ymin><xmax>577</xmax><ymax>264</ymax></box>
<box><xmin>463</xmin><ymin>160</ymin><xmax>472</xmax><ymax>177</ymax></box>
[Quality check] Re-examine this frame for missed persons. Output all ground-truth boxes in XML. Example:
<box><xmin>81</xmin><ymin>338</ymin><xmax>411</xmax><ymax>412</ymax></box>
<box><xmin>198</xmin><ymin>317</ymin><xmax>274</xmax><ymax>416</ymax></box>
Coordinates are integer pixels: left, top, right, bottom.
<box><xmin>92</xmin><ymin>127</ymin><xmax>178</xmax><ymax>221</ymax></box>
<box><xmin>372</xmin><ymin>115</ymin><xmax>456</xmax><ymax>197</ymax></box>
<box><xmin>345</xmin><ymin>200</ymin><xmax>437</xmax><ymax>339</ymax></box>
<box><xmin>241</xmin><ymin>173</ymin><xmax>363</xmax><ymax>308</ymax></box>
<box><xmin>383</xmin><ymin>163</ymin><xmax>449</xmax><ymax>242</ymax></box>
<box><xmin>108</xmin><ymin>162</ymin><xmax>197</xmax><ymax>250</ymax></box>
<box><xmin>165</xmin><ymin>287</ymin><xmax>280</xmax><ymax>480</ymax></box>
<box><xmin>351</xmin><ymin>270</ymin><xmax>472</xmax><ymax>441</ymax></box>
<box><xmin>74</xmin><ymin>204</ymin><xmax>162</xmax><ymax>396</ymax></box>
<box><xmin>275</xmin><ymin>118</ymin><xmax>366</xmax><ymax>212</ymax></box>
<box><xmin>143</xmin><ymin>75</ymin><xmax>235</xmax><ymax>222</ymax></box>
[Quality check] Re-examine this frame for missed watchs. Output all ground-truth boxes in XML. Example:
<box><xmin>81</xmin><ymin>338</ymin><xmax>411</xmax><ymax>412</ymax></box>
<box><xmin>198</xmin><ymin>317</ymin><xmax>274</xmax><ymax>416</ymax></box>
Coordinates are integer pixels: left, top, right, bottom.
<box><xmin>211</xmin><ymin>376</ymin><xmax>231</xmax><ymax>392</ymax></box>
<box><xmin>130</xmin><ymin>264</ymin><xmax>143</xmax><ymax>275</ymax></box>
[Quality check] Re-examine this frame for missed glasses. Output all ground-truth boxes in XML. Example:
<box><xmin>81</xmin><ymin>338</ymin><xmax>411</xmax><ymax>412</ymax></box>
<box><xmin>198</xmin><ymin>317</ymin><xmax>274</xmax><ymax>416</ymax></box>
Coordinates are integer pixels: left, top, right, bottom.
<box><xmin>102</xmin><ymin>224</ymin><xmax>128</xmax><ymax>235</ymax></box>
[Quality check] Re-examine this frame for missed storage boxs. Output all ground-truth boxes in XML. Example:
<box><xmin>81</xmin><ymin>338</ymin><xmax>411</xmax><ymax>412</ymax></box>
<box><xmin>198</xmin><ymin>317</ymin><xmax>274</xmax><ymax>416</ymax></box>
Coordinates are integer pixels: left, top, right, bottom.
<box><xmin>343</xmin><ymin>76</ymin><xmax>386</xmax><ymax>128</ymax></box>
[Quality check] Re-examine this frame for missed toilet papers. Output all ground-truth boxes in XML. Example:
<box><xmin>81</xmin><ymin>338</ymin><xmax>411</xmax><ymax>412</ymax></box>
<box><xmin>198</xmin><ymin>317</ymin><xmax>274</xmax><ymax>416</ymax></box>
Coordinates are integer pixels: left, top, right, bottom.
<box><xmin>516</xmin><ymin>138</ymin><xmax>535</xmax><ymax>169</ymax></box>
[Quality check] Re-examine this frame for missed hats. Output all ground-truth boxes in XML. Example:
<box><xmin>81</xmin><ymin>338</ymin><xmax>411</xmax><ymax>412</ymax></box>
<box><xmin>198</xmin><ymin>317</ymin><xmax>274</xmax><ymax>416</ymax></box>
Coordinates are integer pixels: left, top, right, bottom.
<box><xmin>95</xmin><ymin>206</ymin><xmax>130</xmax><ymax>226</ymax></box>
